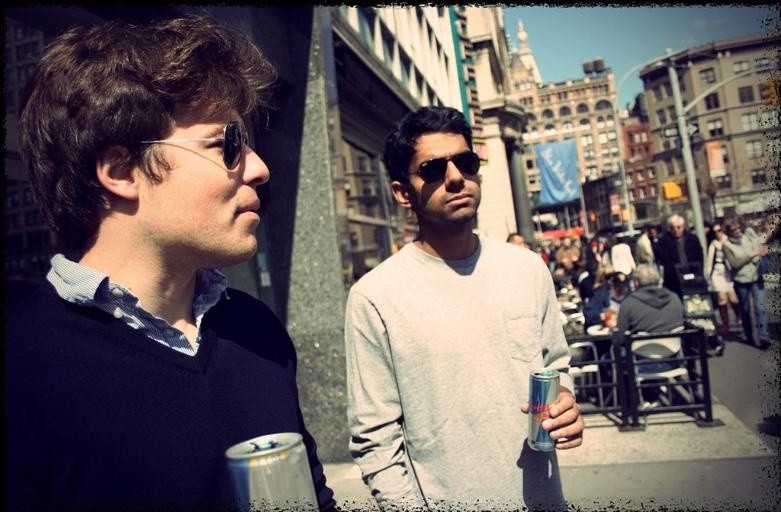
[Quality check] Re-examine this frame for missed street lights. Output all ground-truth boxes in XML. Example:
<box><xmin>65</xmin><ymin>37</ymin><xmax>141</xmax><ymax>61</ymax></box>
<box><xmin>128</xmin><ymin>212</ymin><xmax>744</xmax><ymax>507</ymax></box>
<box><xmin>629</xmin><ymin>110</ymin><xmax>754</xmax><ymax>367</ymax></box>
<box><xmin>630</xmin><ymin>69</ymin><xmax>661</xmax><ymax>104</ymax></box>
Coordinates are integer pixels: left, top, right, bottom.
<box><xmin>704</xmin><ymin>176</ymin><xmax>718</xmax><ymax>219</ymax></box>
<box><xmin>610</xmin><ymin>46</ymin><xmax>690</xmax><ymax>240</ymax></box>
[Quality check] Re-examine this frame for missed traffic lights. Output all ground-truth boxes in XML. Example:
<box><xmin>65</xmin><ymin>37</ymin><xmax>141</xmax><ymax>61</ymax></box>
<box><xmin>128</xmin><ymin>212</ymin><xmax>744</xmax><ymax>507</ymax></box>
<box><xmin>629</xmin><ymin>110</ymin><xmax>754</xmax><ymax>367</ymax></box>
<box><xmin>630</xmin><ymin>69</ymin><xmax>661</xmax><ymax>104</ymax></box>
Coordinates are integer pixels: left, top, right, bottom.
<box><xmin>760</xmin><ymin>77</ymin><xmax>781</xmax><ymax>107</ymax></box>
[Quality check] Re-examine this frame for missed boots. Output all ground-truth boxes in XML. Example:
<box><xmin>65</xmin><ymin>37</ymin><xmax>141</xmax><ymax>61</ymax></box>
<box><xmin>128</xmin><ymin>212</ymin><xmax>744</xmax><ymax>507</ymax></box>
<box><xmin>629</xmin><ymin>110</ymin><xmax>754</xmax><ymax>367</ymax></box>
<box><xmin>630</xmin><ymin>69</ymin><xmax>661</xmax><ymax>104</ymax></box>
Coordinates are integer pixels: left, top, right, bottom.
<box><xmin>730</xmin><ymin>302</ymin><xmax>742</xmax><ymax>325</ymax></box>
<box><xmin>718</xmin><ymin>305</ymin><xmax>729</xmax><ymax>337</ymax></box>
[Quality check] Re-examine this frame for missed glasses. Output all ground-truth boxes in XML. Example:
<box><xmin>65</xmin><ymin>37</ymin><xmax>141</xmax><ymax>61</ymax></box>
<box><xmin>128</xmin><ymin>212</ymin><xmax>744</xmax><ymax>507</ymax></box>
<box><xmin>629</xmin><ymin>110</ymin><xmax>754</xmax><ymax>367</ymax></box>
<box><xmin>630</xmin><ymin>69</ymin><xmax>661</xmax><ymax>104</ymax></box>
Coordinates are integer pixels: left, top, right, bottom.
<box><xmin>405</xmin><ymin>151</ymin><xmax>480</xmax><ymax>185</ymax></box>
<box><xmin>140</xmin><ymin>120</ymin><xmax>250</xmax><ymax>170</ymax></box>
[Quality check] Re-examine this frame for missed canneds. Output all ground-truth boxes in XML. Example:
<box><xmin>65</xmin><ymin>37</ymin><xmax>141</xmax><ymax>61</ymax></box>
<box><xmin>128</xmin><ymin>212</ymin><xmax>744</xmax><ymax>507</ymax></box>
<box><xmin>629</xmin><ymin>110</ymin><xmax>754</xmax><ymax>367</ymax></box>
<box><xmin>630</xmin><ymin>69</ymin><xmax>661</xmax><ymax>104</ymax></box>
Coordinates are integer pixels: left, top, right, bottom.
<box><xmin>528</xmin><ymin>369</ymin><xmax>559</xmax><ymax>453</ymax></box>
<box><xmin>226</xmin><ymin>432</ymin><xmax>319</xmax><ymax>512</ymax></box>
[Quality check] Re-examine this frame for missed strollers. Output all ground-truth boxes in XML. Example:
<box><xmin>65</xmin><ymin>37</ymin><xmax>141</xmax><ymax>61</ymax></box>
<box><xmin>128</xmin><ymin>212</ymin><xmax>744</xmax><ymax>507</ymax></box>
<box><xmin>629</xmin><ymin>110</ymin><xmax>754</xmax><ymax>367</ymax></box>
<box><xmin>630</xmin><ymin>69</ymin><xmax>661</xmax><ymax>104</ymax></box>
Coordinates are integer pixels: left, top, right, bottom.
<box><xmin>673</xmin><ymin>260</ymin><xmax>725</xmax><ymax>359</ymax></box>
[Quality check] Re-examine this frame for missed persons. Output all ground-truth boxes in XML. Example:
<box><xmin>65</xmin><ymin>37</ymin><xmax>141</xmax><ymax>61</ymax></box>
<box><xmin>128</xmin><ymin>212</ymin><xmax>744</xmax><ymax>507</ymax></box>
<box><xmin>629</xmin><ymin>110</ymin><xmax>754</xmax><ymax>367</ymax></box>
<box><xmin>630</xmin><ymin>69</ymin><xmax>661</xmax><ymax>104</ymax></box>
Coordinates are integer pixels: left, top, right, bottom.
<box><xmin>1</xmin><ymin>10</ymin><xmax>341</xmax><ymax>512</ymax></box>
<box><xmin>506</xmin><ymin>213</ymin><xmax>770</xmax><ymax>410</ymax></box>
<box><xmin>345</xmin><ymin>106</ymin><xmax>584</xmax><ymax>511</ymax></box>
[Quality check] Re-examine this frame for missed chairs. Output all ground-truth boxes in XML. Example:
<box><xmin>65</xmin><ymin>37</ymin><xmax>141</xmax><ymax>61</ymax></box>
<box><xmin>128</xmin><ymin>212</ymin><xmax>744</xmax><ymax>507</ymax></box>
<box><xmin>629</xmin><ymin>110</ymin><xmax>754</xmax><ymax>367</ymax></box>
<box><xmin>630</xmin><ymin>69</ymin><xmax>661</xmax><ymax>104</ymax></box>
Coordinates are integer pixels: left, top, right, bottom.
<box><xmin>565</xmin><ymin>326</ymin><xmax>714</xmax><ymax>426</ymax></box>
